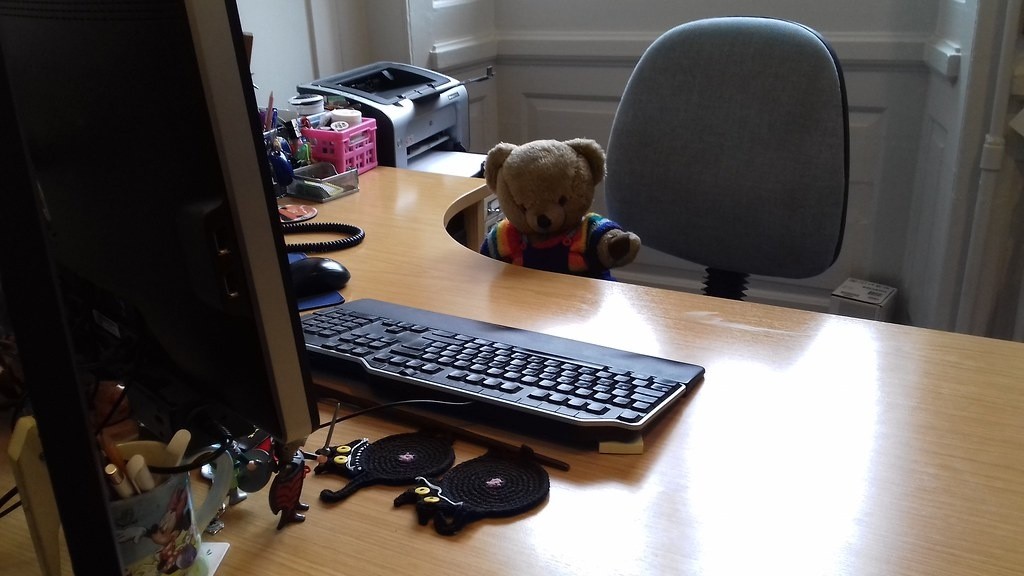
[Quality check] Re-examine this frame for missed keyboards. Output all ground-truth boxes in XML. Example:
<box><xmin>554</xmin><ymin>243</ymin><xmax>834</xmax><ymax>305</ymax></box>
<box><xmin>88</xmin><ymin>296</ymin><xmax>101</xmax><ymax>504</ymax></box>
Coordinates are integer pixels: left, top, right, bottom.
<box><xmin>298</xmin><ymin>298</ymin><xmax>706</xmax><ymax>455</ymax></box>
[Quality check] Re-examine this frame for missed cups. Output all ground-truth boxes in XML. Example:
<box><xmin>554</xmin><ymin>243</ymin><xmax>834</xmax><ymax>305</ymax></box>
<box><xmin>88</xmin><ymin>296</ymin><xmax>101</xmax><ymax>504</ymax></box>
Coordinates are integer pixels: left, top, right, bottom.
<box><xmin>287</xmin><ymin>93</ymin><xmax>324</xmax><ymax>128</ymax></box>
<box><xmin>332</xmin><ymin>109</ymin><xmax>361</xmax><ymax>142</ymax></box>
<box><xmin>98</xmin><ymin>440</ymin><xmax>234</xmax><ymax>576</ymax></box>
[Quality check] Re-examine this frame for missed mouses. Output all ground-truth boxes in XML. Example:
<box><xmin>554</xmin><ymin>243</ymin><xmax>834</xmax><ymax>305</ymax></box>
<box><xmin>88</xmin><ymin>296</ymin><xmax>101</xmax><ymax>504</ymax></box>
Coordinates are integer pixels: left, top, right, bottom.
<box><xmin>290</xmin><ymin>257</ymin><xmax>351</xmax><ymax>300</ymax></box>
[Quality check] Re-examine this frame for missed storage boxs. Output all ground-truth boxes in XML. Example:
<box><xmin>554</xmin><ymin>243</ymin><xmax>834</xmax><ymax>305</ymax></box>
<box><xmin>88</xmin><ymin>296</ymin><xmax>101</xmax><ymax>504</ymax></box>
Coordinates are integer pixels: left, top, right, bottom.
<box><xmin>829</xmin><ymin>279</ymin><xmax>898</xmax><ymax>323</ymax></box>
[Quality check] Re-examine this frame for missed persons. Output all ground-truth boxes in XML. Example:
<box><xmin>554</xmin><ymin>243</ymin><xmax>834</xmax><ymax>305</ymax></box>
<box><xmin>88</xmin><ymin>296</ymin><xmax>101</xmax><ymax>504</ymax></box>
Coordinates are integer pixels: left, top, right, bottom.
<box><xmin>211</xmin><ymin>439</ymin><xmax>257</xmax><ymax>509</ymax></box>
<box><xmin>271</xmin><ymin>438</ymin><xmax>328</xmax><ymax>529</ymax></box>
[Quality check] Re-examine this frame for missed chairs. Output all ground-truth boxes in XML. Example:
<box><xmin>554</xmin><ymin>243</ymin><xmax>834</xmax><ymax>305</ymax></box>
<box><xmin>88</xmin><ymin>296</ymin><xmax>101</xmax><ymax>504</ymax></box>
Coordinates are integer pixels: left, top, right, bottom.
<box><xmin>605</xmin><ymin>16</ymin><xmax>851</xmax><ymax>301</ymax></box>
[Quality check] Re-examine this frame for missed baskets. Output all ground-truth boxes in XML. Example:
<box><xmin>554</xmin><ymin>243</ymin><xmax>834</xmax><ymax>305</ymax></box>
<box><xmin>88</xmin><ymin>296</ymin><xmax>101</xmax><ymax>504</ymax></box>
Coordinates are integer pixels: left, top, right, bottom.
<box><xmin>290</xmin><ymin>117</ymin><xmax>378</xmax><ymax>176</ymax></box>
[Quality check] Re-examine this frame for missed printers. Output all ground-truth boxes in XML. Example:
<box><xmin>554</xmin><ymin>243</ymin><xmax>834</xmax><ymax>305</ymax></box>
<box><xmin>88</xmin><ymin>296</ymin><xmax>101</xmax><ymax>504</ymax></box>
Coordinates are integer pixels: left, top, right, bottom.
<box><xmin>295</xmin><ymin>61</ymin><xmax>497</xmax><ymax>168</ymax></box>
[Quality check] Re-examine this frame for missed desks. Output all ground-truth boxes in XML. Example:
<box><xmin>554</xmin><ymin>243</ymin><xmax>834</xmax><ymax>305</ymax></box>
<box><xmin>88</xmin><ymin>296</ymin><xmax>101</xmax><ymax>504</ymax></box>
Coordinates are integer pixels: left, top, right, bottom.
<box><xmin>0</xmin><ymin>167</ymin><xmax>1024</xmax><ymax>576</ymax></box>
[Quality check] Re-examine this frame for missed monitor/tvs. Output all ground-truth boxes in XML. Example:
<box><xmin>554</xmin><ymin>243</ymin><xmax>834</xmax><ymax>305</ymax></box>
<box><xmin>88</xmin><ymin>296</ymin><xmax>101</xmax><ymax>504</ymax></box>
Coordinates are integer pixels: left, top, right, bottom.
<box><xmin>0</xmin><ymin>1</ymin><xmax>321</xmax><ymax>576</ymax></box>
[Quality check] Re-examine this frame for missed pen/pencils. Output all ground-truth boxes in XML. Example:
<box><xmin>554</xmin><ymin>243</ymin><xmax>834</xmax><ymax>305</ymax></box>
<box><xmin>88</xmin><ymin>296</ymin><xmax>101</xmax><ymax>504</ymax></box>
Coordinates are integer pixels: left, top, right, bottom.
<box><xmin>104</xmin><ymin>455</ymin><xmax>157</xmax><ymax>499</ymax></box>
<box><xmin>260</xmin><ymin>91</ymin><xmax>278</xmax><ymax>130</ymax></box>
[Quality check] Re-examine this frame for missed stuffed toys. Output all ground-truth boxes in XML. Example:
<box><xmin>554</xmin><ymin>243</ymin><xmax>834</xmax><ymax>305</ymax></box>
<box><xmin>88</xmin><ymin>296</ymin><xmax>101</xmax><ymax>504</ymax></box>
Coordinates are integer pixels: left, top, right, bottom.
<box><xmin>479</xmin><ymin>135</ymin><xmax>640</xmax><ymax>282</ymax></box>
